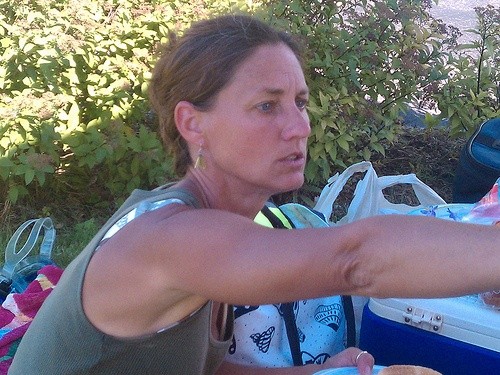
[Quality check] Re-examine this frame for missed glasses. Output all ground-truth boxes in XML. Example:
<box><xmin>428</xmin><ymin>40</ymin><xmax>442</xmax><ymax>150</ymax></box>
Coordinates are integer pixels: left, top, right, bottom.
<box><xmin>0</xmin><ymin>218</ymin><xmax>63</xmax><ymax>308</ymax></box>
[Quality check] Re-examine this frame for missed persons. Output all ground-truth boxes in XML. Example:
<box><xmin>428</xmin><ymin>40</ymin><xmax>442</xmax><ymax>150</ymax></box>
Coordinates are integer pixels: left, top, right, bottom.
<box><xmin>8</xmin><ymin>15</ymin><xmax>500</xmax><ymax>375</ymax></box>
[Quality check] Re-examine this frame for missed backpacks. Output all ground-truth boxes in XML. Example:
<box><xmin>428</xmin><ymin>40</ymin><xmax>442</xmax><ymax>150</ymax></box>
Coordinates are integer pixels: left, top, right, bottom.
<box><xmin>225</xmin><ymin>203</ymin><xmax>355</xmax><ymax>367</ymax></box>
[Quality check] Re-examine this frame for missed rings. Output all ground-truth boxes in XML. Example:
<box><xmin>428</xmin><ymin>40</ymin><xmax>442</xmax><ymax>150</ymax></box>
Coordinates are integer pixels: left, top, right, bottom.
<box><xmin>355</xmin><ymin>351</ymin><xmax>367</xmax><ymax>362</ymax></box>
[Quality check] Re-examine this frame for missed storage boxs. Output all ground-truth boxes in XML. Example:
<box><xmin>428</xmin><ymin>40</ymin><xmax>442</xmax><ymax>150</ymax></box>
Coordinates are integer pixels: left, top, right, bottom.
<box><xmin>359</xmin><ymin>295</ymin><xmax>500</xmax><ymax>374</ymax></box>
